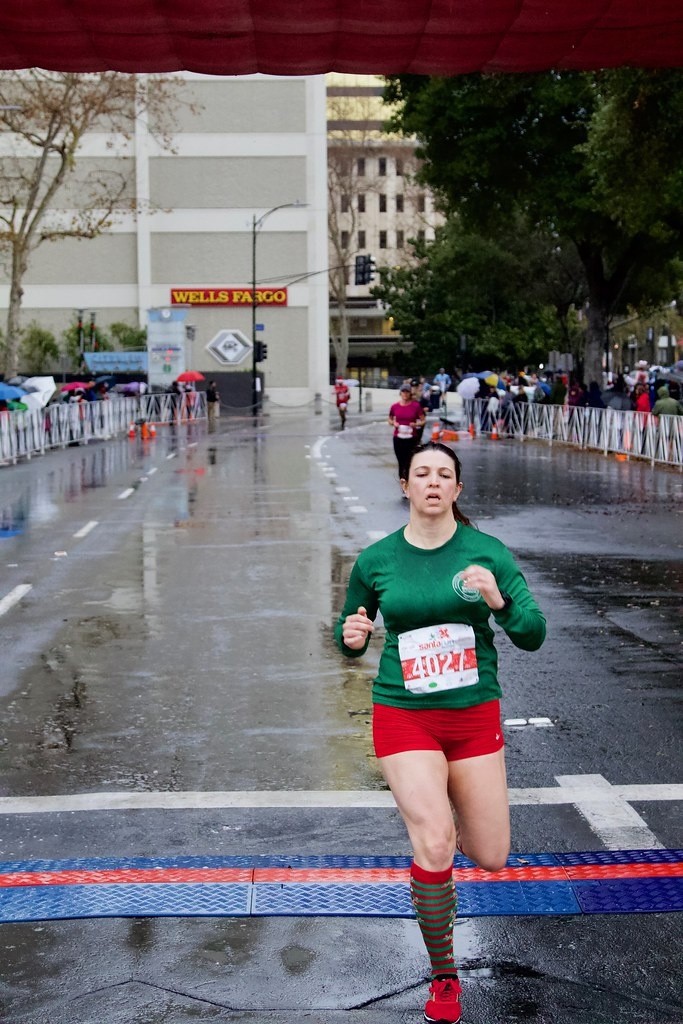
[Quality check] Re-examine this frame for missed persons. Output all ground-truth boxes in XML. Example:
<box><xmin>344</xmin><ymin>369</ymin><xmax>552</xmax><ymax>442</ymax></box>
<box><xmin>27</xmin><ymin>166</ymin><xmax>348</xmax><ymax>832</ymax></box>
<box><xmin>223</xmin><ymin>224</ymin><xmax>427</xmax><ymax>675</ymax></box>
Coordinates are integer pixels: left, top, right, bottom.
<box><xmin>332</xmin><ymin>377</ymin><xmax>352</xmax><ymax>431</ymax></box>
<box><xmin>334</xmin><ymin>441</ymin><xmax>547</xmax><ymax>1024</ymax></box>
<box><xmin>476</xmin><ymin>369</ymin><xmax>683</xmax><ymax>465</ymax></box>
<box><xmin>0</xmin><ymin>382</ymin><xmax>220</xmax><ymax>467</ymax></box>
<box><xmin>388</xmin><ymin>367</ymin><xmax>453</xmax><ymax>501</ymax></box>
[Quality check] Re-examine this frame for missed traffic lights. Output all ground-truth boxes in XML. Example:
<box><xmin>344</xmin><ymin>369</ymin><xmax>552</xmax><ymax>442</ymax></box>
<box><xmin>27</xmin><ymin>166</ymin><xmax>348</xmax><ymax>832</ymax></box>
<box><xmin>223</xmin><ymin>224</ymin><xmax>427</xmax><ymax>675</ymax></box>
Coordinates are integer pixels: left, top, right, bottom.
<box><xmin>355</xmin><ymin>253</ymin><xmax>375</xmax><ymax>283</ymax></box>
<box><xmin>255</xmin><ymin>340</ymin><xmax>267</xmax><ymax>363</ymax></box>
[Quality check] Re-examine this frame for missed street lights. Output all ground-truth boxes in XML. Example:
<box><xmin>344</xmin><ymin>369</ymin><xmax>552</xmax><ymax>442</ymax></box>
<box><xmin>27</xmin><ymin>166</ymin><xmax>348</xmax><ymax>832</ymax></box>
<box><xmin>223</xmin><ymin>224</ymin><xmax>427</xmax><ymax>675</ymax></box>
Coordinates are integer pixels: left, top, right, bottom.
<box><xmin>251</xmin><ymin>203</ymin><xmax>308</xmax><ymax>416</ymax></box>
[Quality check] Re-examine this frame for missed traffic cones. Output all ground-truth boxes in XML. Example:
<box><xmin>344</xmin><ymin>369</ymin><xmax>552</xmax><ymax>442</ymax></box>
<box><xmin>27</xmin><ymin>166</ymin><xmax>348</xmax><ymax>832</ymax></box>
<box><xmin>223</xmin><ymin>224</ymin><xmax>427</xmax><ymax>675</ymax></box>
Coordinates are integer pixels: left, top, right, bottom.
<box><xmin>141</xmin><ymin>422</ymin><xmax>156</xmax><ymax>439</ymax></box>
<box><xmin>129</xmin><ymin>421</ymin><xmax>136</xmax><ymax>436</ymax></box>
<box><xmin>431</xmin><ymin>422</ymin><xmax>440</xmax><ymax>441</ymax></box>
<box><xmin>490</xmin><ymin>424</ymin><xmax>499</xmax><ymax>439</ymax></box>
<box><xmin>467</xmin><ymin>423</ymin><xmax>475</xmax><ymax>438</ymax></box>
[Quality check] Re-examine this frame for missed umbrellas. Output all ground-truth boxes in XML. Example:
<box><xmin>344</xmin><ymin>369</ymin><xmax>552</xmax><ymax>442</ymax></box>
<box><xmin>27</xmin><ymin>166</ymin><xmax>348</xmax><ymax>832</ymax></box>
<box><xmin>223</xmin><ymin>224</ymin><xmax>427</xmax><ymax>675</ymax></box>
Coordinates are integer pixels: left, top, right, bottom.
<box><xmin>651</xmin><ymin>361</ymin><xmax>683</xmax><ymax>384</ymax></box>
<box><xmin>0</xmin><ymin>371</ymin><xmax>206</xmax><ymax>415</ymax></box>
<box><xmin>456</xmin><ymin>370</ymin><xmax>506</xmax><ymax>402</ymax></box>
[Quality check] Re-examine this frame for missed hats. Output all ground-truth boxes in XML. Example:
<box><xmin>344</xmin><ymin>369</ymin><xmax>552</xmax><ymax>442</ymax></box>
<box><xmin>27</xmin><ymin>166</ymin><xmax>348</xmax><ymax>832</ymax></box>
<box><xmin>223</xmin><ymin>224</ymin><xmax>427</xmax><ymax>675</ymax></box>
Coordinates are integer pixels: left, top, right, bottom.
<box><xmin>400</xmin><ymin>384</ymin><xmax>411</xmax><ymax>391</ymax></box>
<box><xmin>635</xmin><ymin>360</ymin><xmax>650</xmax><ymax>369</ymax></box>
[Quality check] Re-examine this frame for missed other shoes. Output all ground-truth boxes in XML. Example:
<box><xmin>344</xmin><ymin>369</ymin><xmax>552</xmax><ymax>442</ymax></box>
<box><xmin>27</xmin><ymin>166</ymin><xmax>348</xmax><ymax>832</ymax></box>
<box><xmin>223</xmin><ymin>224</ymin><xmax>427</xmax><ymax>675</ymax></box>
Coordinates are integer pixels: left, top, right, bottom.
<box><xmin>400</xmin><ymin>488</ymin><xmax>406</xmax><ymax>498</ymax></box>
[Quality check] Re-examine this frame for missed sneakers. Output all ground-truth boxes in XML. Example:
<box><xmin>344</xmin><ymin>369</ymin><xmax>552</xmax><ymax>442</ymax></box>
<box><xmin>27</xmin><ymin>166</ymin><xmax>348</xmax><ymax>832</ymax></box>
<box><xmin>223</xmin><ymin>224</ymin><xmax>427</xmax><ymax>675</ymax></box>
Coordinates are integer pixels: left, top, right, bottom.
<box><xmin>424</xmin><ymin>974</ymin><xmax>463</xmax><ymax>1023</ymax></box>
